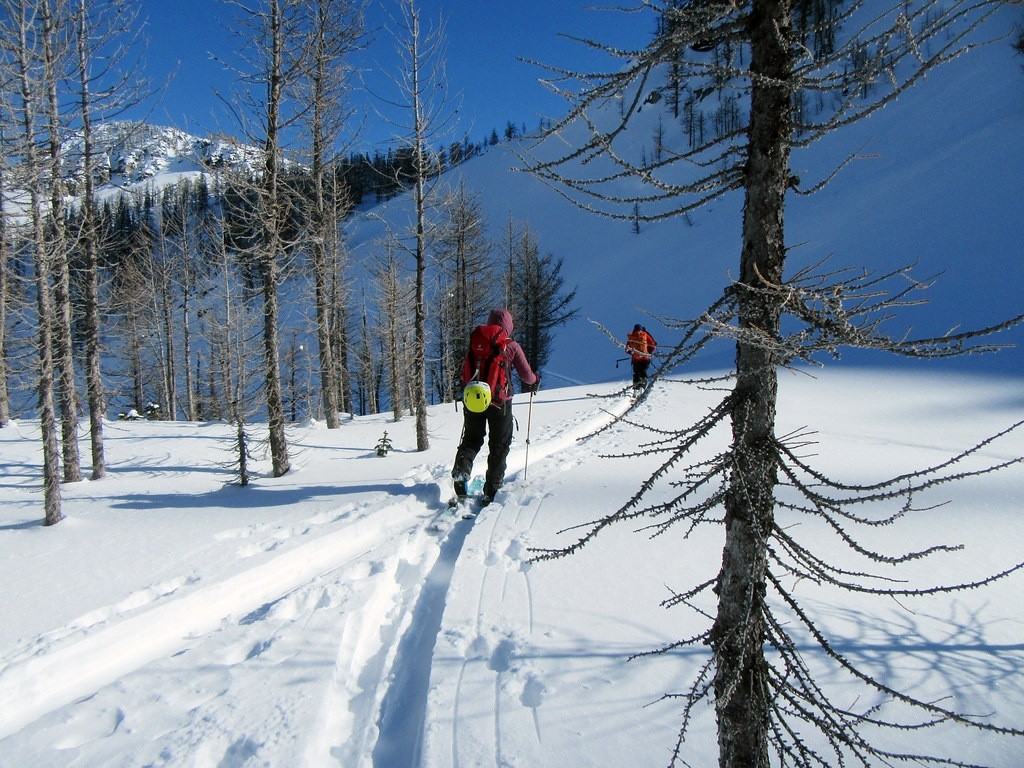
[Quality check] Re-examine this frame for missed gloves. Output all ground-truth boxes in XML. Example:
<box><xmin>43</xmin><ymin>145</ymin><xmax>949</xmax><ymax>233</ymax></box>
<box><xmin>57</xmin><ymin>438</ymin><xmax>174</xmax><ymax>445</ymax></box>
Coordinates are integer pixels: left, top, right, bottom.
<box><xmin>527</xmin><ymin>373</ymin><xmax>539</xmax><ymax>392</ymax></box>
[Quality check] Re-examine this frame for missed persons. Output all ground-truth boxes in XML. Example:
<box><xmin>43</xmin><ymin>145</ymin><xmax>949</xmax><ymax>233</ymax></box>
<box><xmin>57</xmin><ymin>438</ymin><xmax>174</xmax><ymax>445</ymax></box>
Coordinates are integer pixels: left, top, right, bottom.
<box><xmin>625</xmin><ymin>324</ymin><xmax>656</xmax><ymax>399</ymax></box>
<box><xmin>451</xmin><ymin>309</ymin><xmax>540</xmax><ymax>500</ymax></box>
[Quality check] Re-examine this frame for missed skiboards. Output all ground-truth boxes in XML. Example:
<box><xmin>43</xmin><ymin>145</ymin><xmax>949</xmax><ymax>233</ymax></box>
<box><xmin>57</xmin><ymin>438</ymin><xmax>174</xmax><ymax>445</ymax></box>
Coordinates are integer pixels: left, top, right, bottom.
<box><xmin>430</xmin><ymin>474</ymin><xmax>486</xmax><ymax>531</ymax></box>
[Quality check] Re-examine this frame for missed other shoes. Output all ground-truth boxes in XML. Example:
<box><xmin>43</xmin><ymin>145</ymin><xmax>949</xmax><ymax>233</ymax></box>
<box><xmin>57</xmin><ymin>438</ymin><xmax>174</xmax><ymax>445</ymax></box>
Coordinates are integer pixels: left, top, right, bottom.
<box><xmin>484</xmin><ymin>488</ymin><xmax>493</xmax><ymax>507</ymax></box>
<box><xmin>453</xmin><ymin>470</ymin><xmax>468</xmax><ymax>497</ymax></box>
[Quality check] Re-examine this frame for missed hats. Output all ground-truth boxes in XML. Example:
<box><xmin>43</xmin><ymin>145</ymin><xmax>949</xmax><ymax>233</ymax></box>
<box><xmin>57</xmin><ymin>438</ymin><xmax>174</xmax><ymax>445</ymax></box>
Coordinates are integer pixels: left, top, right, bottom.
<box><xmin>634</xmin><ymin>324</ymin><xmax>642</xmax><ymax>331</ymax></box>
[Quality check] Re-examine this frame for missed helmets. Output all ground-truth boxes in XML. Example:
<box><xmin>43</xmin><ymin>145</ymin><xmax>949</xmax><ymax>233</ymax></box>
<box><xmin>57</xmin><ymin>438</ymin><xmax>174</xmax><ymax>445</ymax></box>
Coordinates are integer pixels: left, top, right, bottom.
<box><xmin>463</xmin><ymin>381</ymin><xmax>492</xmax><ymax>414</ymax></box>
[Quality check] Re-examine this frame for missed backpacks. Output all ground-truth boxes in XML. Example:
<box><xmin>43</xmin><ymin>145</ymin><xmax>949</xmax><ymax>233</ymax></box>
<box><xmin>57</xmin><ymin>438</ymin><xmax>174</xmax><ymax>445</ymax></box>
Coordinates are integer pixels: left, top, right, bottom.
<box><xmin>462</xmin><ymin>325</ymin><xmax>507</xmax><ymax>409</ymax></box>
